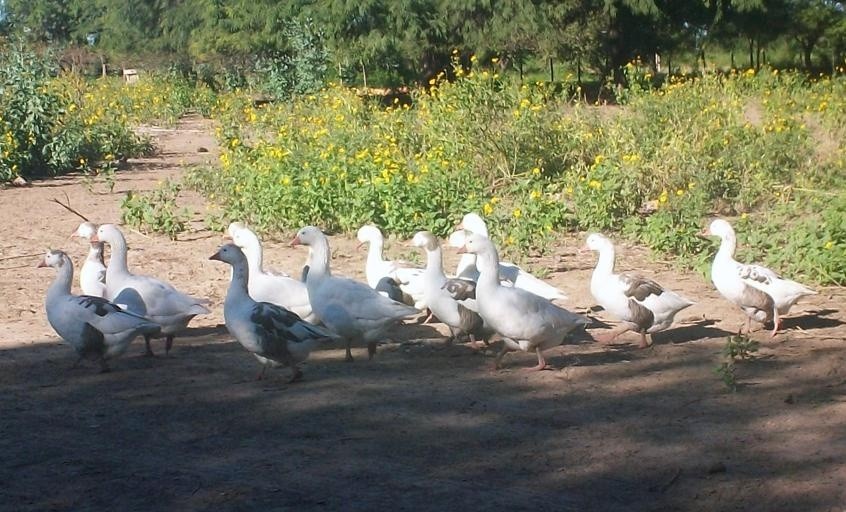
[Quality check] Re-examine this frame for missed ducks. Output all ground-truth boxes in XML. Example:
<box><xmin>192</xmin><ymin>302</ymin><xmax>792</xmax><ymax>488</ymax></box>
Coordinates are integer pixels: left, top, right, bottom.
<box><xmin>701</xmin><ymin>219</ymin><xmax>820</xmax><ymax>338</ymax></box>
<box><xmin>36</xmin><ymin>212</ymin><xmax>594</xmax><ymax>384</ymax></box>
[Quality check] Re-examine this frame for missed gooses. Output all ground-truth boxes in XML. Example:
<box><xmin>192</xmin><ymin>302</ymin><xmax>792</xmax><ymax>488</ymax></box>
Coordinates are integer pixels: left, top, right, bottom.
<box><xmin>578</xmin><ymin>233</ymin><xmax>722</xmax><ymax>350</ymax></box>
